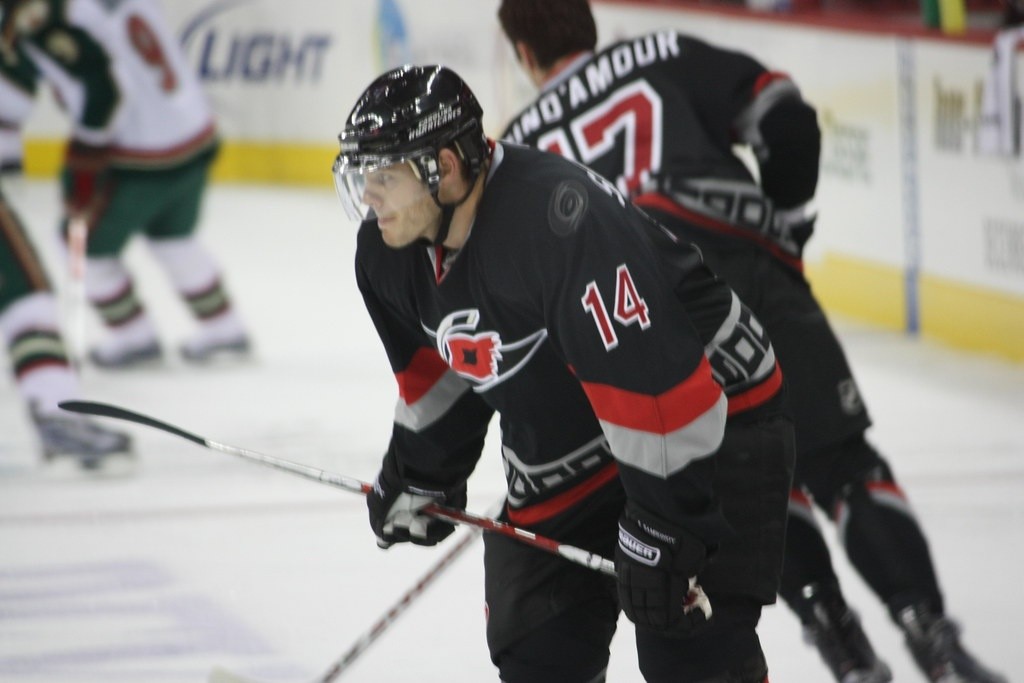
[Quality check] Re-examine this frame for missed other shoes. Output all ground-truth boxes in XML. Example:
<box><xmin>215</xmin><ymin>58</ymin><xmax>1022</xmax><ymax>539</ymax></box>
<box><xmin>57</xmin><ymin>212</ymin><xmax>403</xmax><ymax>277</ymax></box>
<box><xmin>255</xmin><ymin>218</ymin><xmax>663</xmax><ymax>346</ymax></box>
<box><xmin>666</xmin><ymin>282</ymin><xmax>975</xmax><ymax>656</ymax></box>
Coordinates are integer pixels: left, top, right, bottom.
<box><xmin>907</xmin><ymin>620</ymin><xmax>1004</xmax><ymax>682</ymax></box>
<box><xmin>181</xmin><ymin>336</ymin><xmax>250</xmax><ymax>363</ymax></box>
<box><xmin>84</xmin><ymin>340</ymin><xmax>161</xmax><ymax>369</ymax></box>
<box><xmin>814</xmin><ymin>617</ymin><xmax>893</xmax><ymax>681</ymax></box>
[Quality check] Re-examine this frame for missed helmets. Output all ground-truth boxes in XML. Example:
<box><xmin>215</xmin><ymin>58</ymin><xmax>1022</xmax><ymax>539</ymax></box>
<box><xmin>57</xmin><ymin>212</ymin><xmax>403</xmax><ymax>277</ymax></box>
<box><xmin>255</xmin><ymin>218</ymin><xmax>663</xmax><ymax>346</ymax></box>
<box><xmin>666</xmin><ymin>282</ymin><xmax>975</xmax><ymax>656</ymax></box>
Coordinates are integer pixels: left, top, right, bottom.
<box><xmin>332</xmin><ymin>65</ymin><xmax>483</xmax><ymax>166</ymax></box>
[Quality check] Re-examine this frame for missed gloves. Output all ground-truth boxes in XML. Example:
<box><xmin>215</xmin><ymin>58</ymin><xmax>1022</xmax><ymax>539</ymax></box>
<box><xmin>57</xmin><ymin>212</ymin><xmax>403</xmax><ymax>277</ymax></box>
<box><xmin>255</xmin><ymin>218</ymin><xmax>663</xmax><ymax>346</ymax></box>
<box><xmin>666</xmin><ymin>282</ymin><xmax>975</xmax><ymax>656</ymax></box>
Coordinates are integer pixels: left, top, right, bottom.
<box><xmin>613</xmin><ymin>500</ymin><xmax>711</xmax><ymax>637</ymax></box>
<box><xmin>366</xmin><ymin>447</ymin><xmax>467</xmax><ymax>550</ymax></box>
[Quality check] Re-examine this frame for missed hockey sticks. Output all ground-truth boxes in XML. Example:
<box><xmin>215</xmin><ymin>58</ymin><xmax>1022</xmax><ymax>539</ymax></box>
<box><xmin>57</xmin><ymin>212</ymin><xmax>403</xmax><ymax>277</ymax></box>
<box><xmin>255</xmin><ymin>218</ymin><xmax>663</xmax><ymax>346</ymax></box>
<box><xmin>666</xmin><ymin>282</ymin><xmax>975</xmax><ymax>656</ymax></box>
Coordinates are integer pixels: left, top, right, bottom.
<box><xmin>59</xmin><ymin>214</ymin><xmax>90</xmax><ymax>427</ymax></box>
<box><xmin>59</xmin><ymin>400</ymin><xmax>713</xmax><ymax>621</ymax></box>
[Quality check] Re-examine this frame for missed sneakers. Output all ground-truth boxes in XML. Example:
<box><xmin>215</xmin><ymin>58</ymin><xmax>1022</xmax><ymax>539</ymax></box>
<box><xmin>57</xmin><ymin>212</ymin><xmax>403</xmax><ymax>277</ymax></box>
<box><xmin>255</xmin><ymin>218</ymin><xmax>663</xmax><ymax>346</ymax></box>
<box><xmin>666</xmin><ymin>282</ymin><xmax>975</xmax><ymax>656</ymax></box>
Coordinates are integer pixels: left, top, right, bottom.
<box><xmin>32</xmin><ymin>410</ymin><xmax>131</xmax><ymax>471</ymax></box>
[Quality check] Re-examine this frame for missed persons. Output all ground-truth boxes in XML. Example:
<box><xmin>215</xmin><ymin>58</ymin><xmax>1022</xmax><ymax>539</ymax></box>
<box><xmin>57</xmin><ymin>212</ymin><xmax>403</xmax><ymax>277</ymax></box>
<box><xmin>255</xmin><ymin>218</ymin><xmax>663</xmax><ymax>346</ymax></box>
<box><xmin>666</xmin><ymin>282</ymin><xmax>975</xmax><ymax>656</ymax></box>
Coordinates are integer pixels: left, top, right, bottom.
<box><xmin>0</xmin><ymin>0</ymin><xmax>256</xmax><ymax>471</ymax></box>
<box><xmin>496</xmin><ymin>0</ymin><xmax>1010</xmax><ymax>683</ymax></box>
<box><xmin>332</xmin><ymin>64</ymin><xmax>797</xmax><ymax>683</ymax></box>
